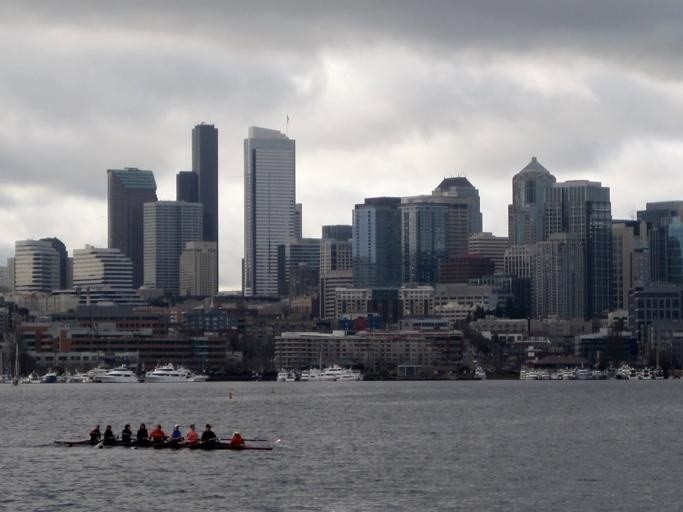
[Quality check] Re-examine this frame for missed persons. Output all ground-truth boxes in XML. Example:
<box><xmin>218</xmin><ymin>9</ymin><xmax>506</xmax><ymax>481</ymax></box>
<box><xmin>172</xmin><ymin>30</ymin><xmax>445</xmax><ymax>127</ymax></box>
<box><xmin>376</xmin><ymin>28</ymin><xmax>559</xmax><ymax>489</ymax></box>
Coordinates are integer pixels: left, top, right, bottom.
<box><xmin>137</xmin><ymin>424</ymin><xmax>148</xmax><ymax>441</ymax></box>
<box><xmin>171</xmin><ymin>424</ymin><xmax>184</xmax><ymax>443</ymax></box>
<box><xmin>149</xmin><ymin>425</ymin><xmax>165</xmax><ymax>443</ymax></box>
<box><xmin>200</xmin><ymin>424</ymin><xmax>216</xmax><ymax>442</ymax></box>
<box><xmin>104</xmin><ymin>425</ymin><xmax>116</xmax><ymax>443</ymax></box>
<box><xmin>122</xmin><ymin>424</ymin><xmax>132</xmax><ymax>442</ymax></box>
<box><xmin>187</xmin><ymin>424</ymin><xmax>198</xmax><ymax>442</ymax></box>
<box><xmin>89</xmin><ymin>424</ymin><xmax>101</xmax><ymax>441</ymax></box>
<box><xmin>230</xmin><ymin>432</ymin><xmax>245</xmax><ymax>447</ymax></box>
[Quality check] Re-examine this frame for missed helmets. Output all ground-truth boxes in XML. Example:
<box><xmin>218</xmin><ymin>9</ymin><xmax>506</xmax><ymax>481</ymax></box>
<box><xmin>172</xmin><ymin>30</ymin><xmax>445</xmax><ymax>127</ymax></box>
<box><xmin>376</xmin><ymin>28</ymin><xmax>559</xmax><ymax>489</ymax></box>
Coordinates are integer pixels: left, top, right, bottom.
<box><xmin>206</xmin><ymin>424</ymin><xmax>212</xmax><ymax>427</ymax></box>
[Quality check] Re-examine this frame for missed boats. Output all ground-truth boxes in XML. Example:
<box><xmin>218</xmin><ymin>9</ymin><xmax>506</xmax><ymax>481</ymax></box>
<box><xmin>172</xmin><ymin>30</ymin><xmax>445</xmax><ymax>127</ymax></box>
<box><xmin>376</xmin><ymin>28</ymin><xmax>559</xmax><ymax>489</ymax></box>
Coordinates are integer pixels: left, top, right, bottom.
<box><xmin>54</xmin><ymin>434</ymin><xmax>273</xmax><ymax>450</ymax></box>
<box><xmin>520</xmin><ymin>363</ymin><xmax>663</xmax><ymax>380</ymax></box>
<box><xmin>277</xmin><ymin>364</ymin><xmax>364</xmax><ymax>382</ymax></box>
<box><xmin>11</xmin><ymin>363</ymin><xmax>209</xmax><ymax>385</ymax></box>
<box><xmin>474</xmin><ymin>366</ymin><xmax>487</xmax><ymax>380</ymax></box>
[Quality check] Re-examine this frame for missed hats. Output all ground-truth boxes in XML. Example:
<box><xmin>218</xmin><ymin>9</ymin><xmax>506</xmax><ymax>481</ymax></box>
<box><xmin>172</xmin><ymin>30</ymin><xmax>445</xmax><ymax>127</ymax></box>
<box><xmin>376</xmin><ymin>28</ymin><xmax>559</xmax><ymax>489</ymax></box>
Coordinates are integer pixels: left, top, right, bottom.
<box><xmin>174</xmin><ymin>424</ymin><xmax>180</xmax><ymax>428</ymax></box>
